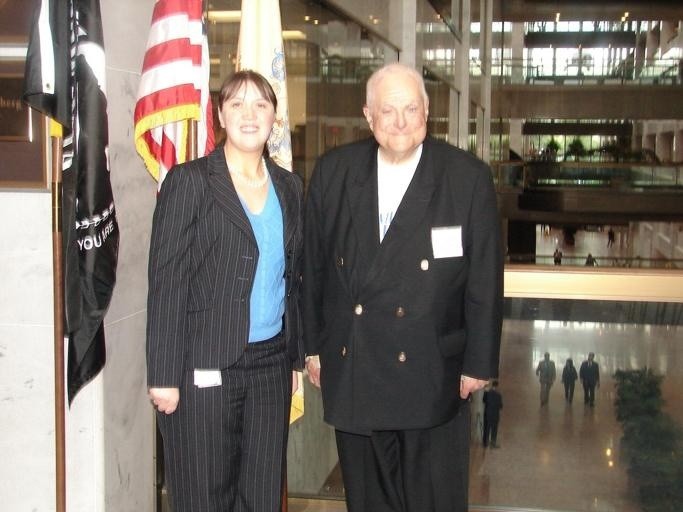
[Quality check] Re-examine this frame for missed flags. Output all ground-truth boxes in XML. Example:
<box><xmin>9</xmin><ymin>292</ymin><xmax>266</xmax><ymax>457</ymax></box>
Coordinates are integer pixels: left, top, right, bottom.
<box><xmin>133</xmin><ymin>0</ymin><xmax>215</xmax><ymax>200</ymax></box>
<box><xmin>236</xmin><ymin>0</ymin><xmax>303</xmax><ymax>425</ymax></box>
<box><xmin>21</xmin><ymin>0</ymin><xmax>119</xmax><ymax>411</ymax></box>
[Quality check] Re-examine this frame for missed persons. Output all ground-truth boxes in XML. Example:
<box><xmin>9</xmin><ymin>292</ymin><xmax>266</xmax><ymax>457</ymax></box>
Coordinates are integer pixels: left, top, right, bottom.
<box><xmin>149</xmin><ymin>66</ymin><xmax>306</xmax><ymax>512</ymax></box>
<box><xmin>482</xmin><ymin>381</ymin><xmax>504</xmax><ymax>448</ymax></box>
<box><xmin>580</xmin><ymin>352</ymin><xmax>600</xmax><ymax>406</ymax></box>
<box><xmin>553</xmin><ymin>250</ymin><xmax>561</xmax><ymax>265</ymax></box>
<box><xmin>605</xmin><ymin>139</ymin><xmax>624</xmax><ymax>163</ymax></box>
<box><xmin>303</xmin><ymin>63</ymin><xmax>507</xmax><ymax>511</ymax></box>
<box><xmin>608</xmin><ymin>228</ymin><xmax>615</xmax><ymax>247</ymax></box>
<box><xmin>585</xmin><ymin>254</ymin><xmax>598</xmax><ymax>267</ymax></box>
<box><xmin>561</xmin><ymin>359</ymin><xmax>578</xmax><ymax>402</ymax></box>
<box><xmin>536</xmin><ymin>352</ymin><xmax>556</xmax><ymax>407</ymax></box>
<box><xmin>600</xmin><ymin>224</ymin><xmax>604</xmax><ymax>232</ymax></box>
